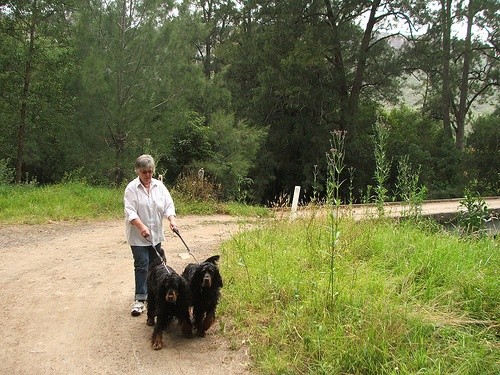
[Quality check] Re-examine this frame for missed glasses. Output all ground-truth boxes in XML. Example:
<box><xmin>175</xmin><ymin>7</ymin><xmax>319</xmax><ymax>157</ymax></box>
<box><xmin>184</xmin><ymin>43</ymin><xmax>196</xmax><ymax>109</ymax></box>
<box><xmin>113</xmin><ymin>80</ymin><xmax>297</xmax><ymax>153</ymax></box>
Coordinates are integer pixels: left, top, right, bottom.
<box><xmin>138</xmin><ymin>169</ymin><xmax>154</xmax><ymax>175</ymax></box>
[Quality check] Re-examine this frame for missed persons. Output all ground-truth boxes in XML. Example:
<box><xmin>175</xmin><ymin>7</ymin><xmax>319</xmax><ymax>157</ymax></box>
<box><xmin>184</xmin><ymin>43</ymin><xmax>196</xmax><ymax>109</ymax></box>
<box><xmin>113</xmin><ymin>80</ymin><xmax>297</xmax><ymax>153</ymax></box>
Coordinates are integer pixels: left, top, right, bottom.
<box><xmin>124</xmin><ymin>154</ymin><xmax>178</xmax><ymax>315</ymax></box>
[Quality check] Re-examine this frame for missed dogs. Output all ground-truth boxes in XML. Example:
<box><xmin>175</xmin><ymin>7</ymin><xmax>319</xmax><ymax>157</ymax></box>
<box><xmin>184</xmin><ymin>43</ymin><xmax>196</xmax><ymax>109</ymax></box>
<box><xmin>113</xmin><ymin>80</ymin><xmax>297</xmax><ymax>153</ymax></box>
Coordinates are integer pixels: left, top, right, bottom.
<box><xmin>146</xmin><ymin>248</ymin><xmax>193</xmax><ymax>350</ymax></box>
<box><xmin>180</xmin><ymin>254</ymin><xmax>223</xmax><ymax>337</ymax></box>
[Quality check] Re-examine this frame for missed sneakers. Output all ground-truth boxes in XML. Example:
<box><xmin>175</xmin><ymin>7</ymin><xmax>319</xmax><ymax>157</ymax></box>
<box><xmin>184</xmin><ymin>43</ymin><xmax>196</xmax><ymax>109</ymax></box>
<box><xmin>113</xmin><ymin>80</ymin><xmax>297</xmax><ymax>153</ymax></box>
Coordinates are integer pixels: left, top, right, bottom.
<box><xmin>129</xmin><ymin>299</ymin><xmax>147</xmax><ymax>318</ymax></box>
